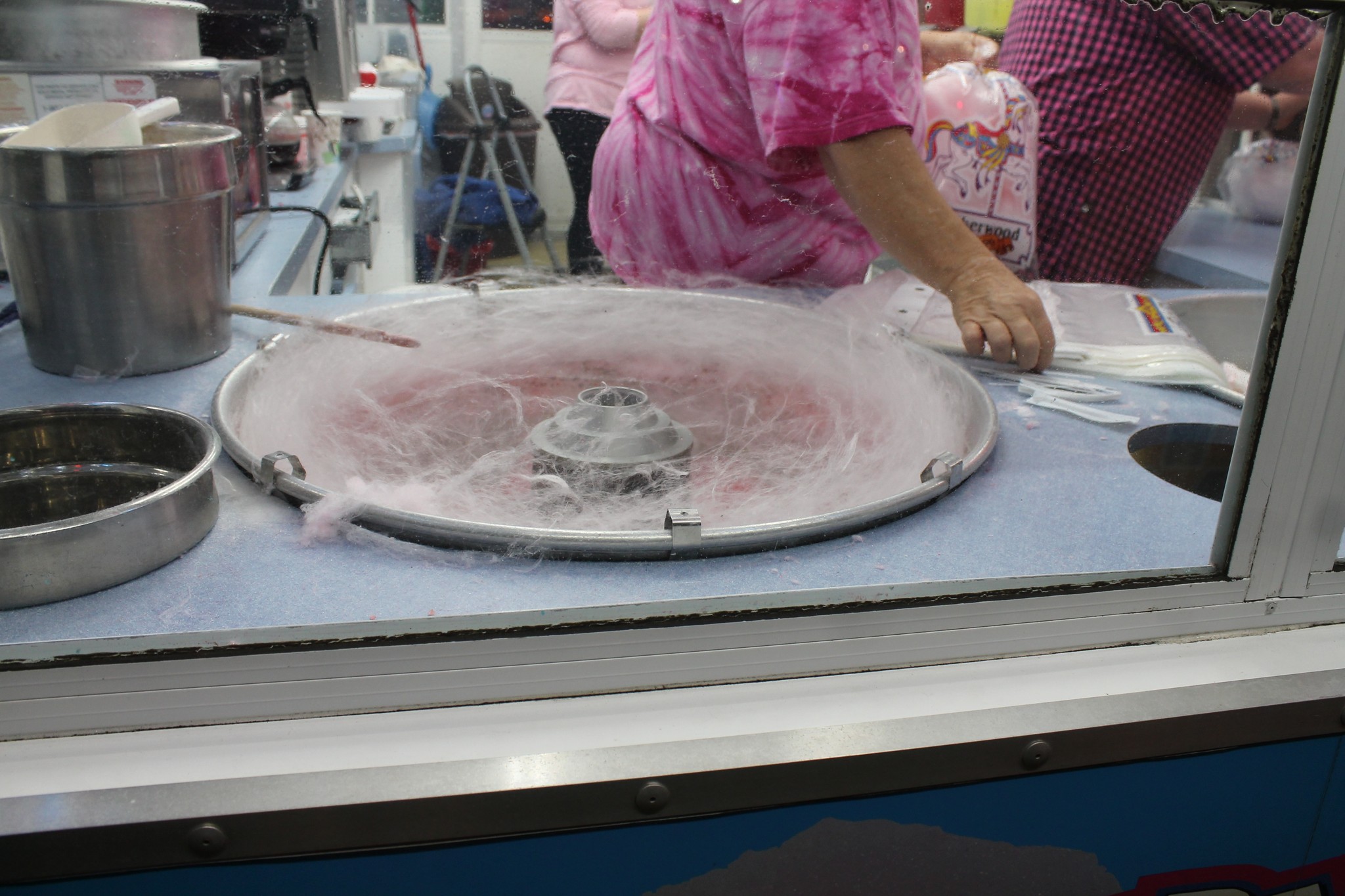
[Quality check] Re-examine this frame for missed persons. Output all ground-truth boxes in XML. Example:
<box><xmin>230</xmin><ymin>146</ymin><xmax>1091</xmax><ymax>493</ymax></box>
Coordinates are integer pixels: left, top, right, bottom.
<box><xmin>991</xmin><ymin>0</ymin><xmax>1328</xmax><ymax>286</ymax></box>
<box><xmin>584</xmin><ymin>0</ymin><xmax>1056</xmax><ymax>373</ymax></box>
<box><xmin>541</xmin><ymin>0</ymin><xmax>655</xmax><ymax>276</ymax></box>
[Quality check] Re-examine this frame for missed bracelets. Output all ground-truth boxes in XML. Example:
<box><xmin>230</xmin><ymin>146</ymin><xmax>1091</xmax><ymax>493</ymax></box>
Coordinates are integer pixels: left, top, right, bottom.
<box><xmin>1264</xmin><ymin>95</ymin><xmax>1280</xmax><ymax>130</ymax></box>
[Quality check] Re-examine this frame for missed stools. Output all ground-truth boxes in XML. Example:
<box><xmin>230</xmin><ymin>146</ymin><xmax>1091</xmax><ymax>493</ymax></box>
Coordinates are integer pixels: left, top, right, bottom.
<box><xmin>432</xmin><ymin>120</ymin><xmax>562</xmax><ymax>288</ymax></box>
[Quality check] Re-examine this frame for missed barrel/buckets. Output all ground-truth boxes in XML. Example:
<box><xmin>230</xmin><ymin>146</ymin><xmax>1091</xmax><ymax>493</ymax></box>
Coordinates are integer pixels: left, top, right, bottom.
<box><xmin>0</xmin><ymin>114</ymin><xmax>242</xmax><ymax>379</ymax></box>
